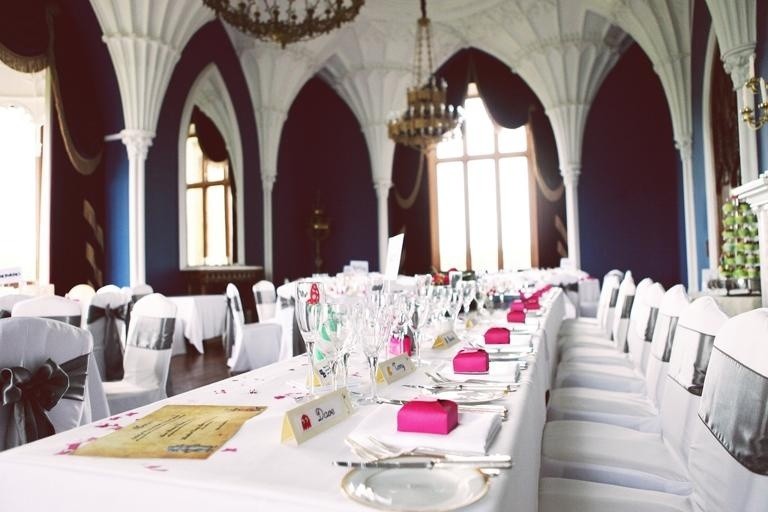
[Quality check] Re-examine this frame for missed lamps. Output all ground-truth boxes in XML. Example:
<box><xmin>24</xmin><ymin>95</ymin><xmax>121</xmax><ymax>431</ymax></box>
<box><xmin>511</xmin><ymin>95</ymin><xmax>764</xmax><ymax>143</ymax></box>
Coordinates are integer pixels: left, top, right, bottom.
<box><xmin>387</xmin><ymin>0</ymin><xmax>465</xmax><ymax>152</ymax></box>
<box><xmin>200</xmin><ymin>1</ymin><xmax>364</xmax><ymax>48</ymax></box>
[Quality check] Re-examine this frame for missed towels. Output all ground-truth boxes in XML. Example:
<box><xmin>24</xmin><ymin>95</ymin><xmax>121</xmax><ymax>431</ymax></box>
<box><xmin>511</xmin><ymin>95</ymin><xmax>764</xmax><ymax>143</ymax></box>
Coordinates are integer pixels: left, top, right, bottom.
<box><xmin>495</xmin><ymin>287</ymin><xmax>561</xmax><ymax>331</ymax></box>
<box><xmin>436</xmin><ymin>359</ymin><xmax>521</xmax><ymax>384</ymax></box>
<box><xmin>343</xmin><ymin>401</ymin><xmax>505</xmax><ymax>465</ymax></box>
<box><xmin>467</xmin><ymin>332</ymin><xmax>533</xmax><ymax>353</ymax></box>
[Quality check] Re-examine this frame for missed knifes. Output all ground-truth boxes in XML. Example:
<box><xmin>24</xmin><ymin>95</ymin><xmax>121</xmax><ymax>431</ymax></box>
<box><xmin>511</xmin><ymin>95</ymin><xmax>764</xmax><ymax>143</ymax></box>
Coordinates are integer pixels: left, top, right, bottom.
<box><xmin>335</xmin><ymin>460</ymin><xmax>513</xmax><ymax>468</ymax></box>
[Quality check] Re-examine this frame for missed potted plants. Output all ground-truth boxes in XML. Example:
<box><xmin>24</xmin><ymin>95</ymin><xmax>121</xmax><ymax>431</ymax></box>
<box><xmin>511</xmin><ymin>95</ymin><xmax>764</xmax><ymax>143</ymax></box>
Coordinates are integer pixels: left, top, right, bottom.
<box><xmin>708</xmin><ymin>194</ymin><xmax>762</xmax><ymax>320</ymax></box>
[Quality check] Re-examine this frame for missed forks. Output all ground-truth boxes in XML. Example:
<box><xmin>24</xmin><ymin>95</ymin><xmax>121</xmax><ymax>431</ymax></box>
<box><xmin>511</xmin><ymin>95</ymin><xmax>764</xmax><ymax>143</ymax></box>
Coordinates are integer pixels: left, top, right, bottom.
<box><xmin>368</xmin><ymin>434</ymin><xmax>512</xmax><ymax>460</ymax></box>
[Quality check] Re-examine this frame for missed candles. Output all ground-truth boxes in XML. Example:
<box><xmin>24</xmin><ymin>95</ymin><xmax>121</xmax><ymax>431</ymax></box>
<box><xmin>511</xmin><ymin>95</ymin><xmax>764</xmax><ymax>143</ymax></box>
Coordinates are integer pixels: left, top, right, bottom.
<box><xmin>748</xmin><ymin>55</ymin><xmax>755</xmax><ymax>81</ymax></box>
<box><xmin>759</xmin><ymin>76</ymin><xmax>767</xmax><ymax>105</ymax></box>
<box><xmin>742</xmin><ymin>82</ymin><xmax>749</xmax><ymax>110</ymax></box>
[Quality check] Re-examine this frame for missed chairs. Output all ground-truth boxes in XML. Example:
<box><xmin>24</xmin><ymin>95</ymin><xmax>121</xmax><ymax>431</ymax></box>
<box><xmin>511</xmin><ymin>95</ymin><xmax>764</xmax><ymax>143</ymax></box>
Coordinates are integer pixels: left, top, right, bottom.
<box><xmin>223</xmin><ymin>283</ymin><xmax>280</xmax><ymax>373</ymax></box>
<box><xmin>558</xmin><ymin>277</ymin><xmax>621</xmax><ymax>343</ymax></box>
<box><xmin>541</xmin><ymin>295</ymin><xmax>732</xmax><ymax>495</ymax></box>
<box><xmin>579</xmin><ymin>269</ymin><xmax>623</xmax><ymax>325</ymax></box>
<box><xmin>539</xmin><ymin>308</ymin><xmax>768</xmax><ymax>511</ymax></box>
<box><xmin>561</xmin><ymin>279</ymin><xmax>656</xmax><ymax>364</ymax></box>
<box><xmin>555</xmin><ymin>282</ymin><xmax>664</xmax><ymax>391</ymax></box>
<box><xmin>131</xmin><ymin>284</ymin><xmax>152</xmax><ymax>298</ymax></box>
<box><xmin>548</xmin><ymin>283</ymin><xmax>692</xmax><ymax>433</ymax></box>
<box><xmin>64</xmin><ymin>284</ymin><xmax>92</xmax><ymax>305</ymax></box>
<box><xmin>97</xmin><ymin>282</ymin><xmax>118</xmax><ymax>294</ymax></box>
<box><xmin>103</xmin><ymin>294</ymin><xmax>177</xmax><ymax>416</ymax></box>
<box><xmin>252</xmin><ymin>280</ymin><xmax>277</xmax><ymax>323</ymax></box>
<box><xmin>86</xmin><ymin>285</ymin><xmax>128</xmax><ymax>382</ymax></box>
<box><xmin>277</xmin><ymin>281</ymin><xmax>295</xmax><ymax>361</ymax></box>
<box><xmin>562</xmin><ymin>277</ymin><xmax>637</xmax><ymax>352</ymax></box>
<box><xmin>0</xmin><ymin>294</ymin><xmax>32</xmax><ymax>318</ymax></box>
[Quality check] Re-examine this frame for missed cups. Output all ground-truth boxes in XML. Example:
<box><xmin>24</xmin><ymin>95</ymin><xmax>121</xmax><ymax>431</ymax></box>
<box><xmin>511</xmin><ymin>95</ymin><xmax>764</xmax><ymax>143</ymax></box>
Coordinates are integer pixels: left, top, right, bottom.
<box><xmin>409</xmin><ymin>296</ymin><xmax>428</xmax><ymax>367</ymax></box>
<box><xmin>435</xmin><ymin>286</ymin><xmax>453</xmax><ymax>326</ymax></box>
<box><xmin>354</xmin><ymin>272</ymin><xmax>388</xmax><ymax>407</ymax></box>
<box><xmin>457</xmin><ymin>280</ymin><xmax>476</xmax><ymax>325</ymax></box>
<box><xmin>387</xmin><ymin>290</ymin><xmax>409</xmax><ymax>354</ymax></box>
<box><xmin>326</xmin><ymin>300</ymin><xmax>352</xmax><ymax>409</ymax></box>
<box><xmin>294</xmin><ymin>280</ymin><xmax>326</xmax><ymax>402</ymax></box>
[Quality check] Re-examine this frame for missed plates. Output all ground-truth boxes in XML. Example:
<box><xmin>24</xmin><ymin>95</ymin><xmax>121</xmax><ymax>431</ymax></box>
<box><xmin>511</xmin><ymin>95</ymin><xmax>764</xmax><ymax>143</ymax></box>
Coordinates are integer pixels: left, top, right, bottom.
<box><xmin>488</xmin><ymin>352</ymin><xmax>522</xmax><ymax>361</ymax></box>
<box><xmin>341</xmin><ymin>448</ymin><xmax>490</xmax><ymax>506</ymax></box>
<box><xmin>436</xmin><ymin>389</ymin><xmax>507</xmax><ymax>404</ymax></box>
